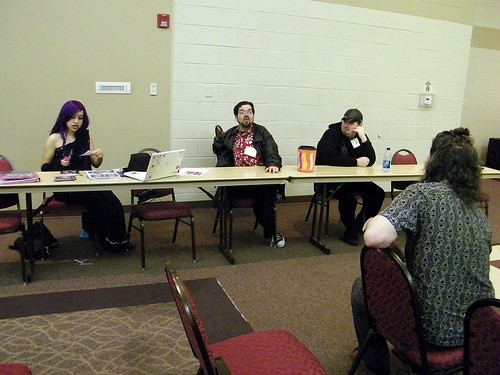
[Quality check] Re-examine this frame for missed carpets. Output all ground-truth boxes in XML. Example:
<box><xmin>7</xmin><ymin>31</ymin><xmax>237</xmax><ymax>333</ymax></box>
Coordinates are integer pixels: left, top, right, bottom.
<box><xmin>0</xmin><ymin>278</ymin><xmax>254</xmax><ymax>375</ymax></box>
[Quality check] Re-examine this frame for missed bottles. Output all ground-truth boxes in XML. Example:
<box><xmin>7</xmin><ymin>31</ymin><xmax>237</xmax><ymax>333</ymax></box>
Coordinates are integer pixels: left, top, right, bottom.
<box><xmin>382</xmin><ymin>147</ymin><xmax>391</xmax><ymax>172</ymax></box>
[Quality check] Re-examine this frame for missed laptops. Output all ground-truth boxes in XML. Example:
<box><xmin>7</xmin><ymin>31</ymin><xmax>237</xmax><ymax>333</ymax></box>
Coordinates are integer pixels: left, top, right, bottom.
<box><xmin>123</xmin><ymin>149</ymin><xmax>186</xmax><ymax>182</ymax></box>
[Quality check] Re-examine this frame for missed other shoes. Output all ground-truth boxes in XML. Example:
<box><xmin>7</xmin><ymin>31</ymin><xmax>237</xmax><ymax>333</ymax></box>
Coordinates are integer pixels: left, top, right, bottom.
<box><xmin>344</xmin><ymin>228</ymin><xmax>364</xmax><ymax>246</ymax></box>
<box><xmin>104</xmin><ymin>240</ymin><xmax>136</xmax><ymax>253</ymax></box>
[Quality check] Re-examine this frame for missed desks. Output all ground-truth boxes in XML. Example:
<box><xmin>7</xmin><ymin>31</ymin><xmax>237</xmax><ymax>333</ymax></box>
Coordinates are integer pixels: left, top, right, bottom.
<box><xmin>0</xmin><ymin>165</ymin><xmax>500</xmax><ymax>282</ymax></box>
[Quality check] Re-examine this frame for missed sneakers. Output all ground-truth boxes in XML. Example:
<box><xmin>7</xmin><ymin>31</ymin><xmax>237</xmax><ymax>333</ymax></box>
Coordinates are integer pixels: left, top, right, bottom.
<box><xmin>268</xmin><ymin>230</ymin><xmax>286</xmax><ymax>248</ymax></box>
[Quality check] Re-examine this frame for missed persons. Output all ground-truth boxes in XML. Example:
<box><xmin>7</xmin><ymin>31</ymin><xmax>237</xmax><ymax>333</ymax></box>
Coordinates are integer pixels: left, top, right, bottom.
<box><xmin>314</xmin><ymin>109</ymin><xmax>384</xmax><ymax>246</ymax></box>
<box><xmin>351</xmin><ymin>127</ymin><xmax>495</xmax><ymax>375</ymax></box>
<box><xmin>213</xmin><ymin>101</ymin><xmax>285</xmax><ymax>247</ymax></box>
<box><xmin>40</xmin><ymin>100</ymin><xmax>136</xmax><ymax>252</ymax></box>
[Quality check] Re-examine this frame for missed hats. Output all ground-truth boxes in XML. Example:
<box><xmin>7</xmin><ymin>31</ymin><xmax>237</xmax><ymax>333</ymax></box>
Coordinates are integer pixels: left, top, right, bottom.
<box><xmin>342</xmin><ymin>108</ymin><xmax>364</xmax><ymax>124</ymax></box>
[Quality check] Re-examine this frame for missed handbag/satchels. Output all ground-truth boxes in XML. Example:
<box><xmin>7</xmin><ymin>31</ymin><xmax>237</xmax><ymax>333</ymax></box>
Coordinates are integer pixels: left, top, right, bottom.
<box><xmin>7</xmin><ymin>221</ymin><xmax>59</xmax><ymax>262</ymax></box>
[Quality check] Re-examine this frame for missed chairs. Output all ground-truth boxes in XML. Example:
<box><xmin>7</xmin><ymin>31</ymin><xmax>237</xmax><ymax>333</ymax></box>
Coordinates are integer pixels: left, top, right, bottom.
<box><xmin>391</xmin><ymin>149</ymin><xmax>417</xmax><ymax>200</ymax></box>
<box><xmin>40</xmin><ymin>190</ymin><xmax>99</xmax><ymax>257</ymax></box>
<box><xmin>347</xmin><ymin>245</ymin><xmax>500</xmax><ymax>375</ymax></box>
<box><xmin>212</xmin><ymin>191</ymin><xmax>269</xmax><ymax>253</ymax></box>
<box><xmin>305</xmin><ymin>183</ymin><xmax>364</xmax><ymax>237</ymax></box>
<box><xmin>0</xmin><ymin>364</ymin><xmax>32</xmax><ymax>375</ymax></box>
<box><xmin>0</xmin><ymin>155</ymin><xmax>26</xmax><ymax>286</ymax></box>
<box><xmin>478</xmin><ymin>192</ymin><xmax>489</xmax><ymax>217</ymax></box>
<box><xmin>127</xmin><ymin>148</ymin><xmax>195</xmax><ymax>271</ymax></box>
<box><xmin>164</xmin><ymin>261</ymin><xmax>327</xmax><ymax>375</ymax></box>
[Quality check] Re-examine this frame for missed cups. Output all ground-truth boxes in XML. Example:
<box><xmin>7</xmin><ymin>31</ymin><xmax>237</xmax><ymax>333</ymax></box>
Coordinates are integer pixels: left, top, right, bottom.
<box><xmin>297</xmin><ymin>145</ymin><xmax>317</xmax><ymax>174</ymax></box>
